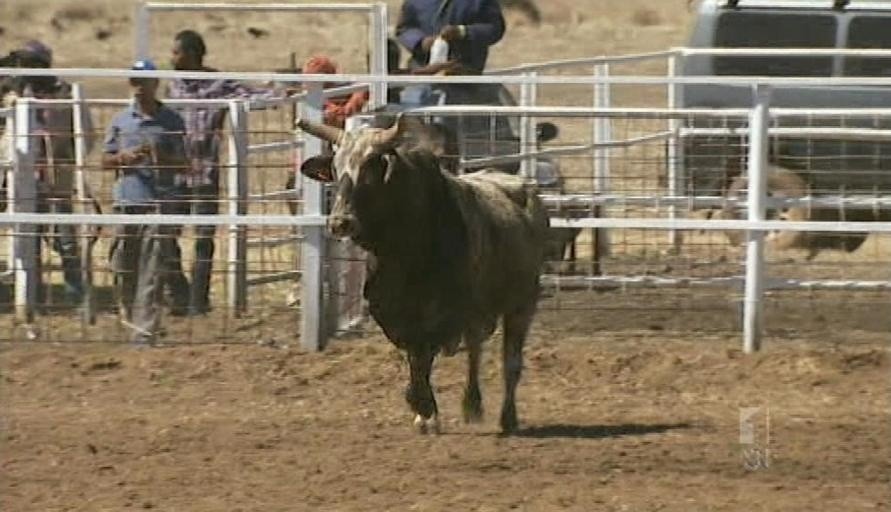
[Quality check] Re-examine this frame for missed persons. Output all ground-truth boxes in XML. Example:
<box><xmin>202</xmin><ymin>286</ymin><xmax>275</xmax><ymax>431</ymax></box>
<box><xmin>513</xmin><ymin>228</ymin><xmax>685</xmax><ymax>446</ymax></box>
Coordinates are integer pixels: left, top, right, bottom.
<box><xmin>158</xmin><ymin>30</ymin><xmax>307</xmax><ymax>318</ymax></box>
<box><xmin>303</xmin><ymin>59</ymin><xmax>370</xmax><ymax>344</ymax></box>
<box><xmin>366</xmin><ymin>35</ymin><xmax>406</xmax><ymax>105</ymax></box>
<box><xmin>0</xmin><ymin>40</ymin><xmax>97</xmax><ymax>319</ymax></box>
<box><xmin>102</xmin><ymin>57</ymin><xmax>191</xmax><ymax>349</ymax></box>
<box><xmin>394</xmin><ymin>0</ymin><xmax>509</xmax><ymax>168</ymax></box>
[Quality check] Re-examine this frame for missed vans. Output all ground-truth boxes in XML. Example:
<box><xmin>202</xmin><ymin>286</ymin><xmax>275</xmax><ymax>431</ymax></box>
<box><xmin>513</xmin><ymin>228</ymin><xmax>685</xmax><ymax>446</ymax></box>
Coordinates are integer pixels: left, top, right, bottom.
<box><xmin>649</xmin><ymin>0</ymin><xmax>891</xmax><ymax>258</ymax></box>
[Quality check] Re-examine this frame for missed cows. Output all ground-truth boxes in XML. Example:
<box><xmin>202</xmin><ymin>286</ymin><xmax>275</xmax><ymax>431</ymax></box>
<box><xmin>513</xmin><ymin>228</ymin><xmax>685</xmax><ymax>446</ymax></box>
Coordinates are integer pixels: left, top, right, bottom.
<box><xmin>293</xmin><ymin>111</ymin><xmax>550</xmax><ymax>436</ymax></box>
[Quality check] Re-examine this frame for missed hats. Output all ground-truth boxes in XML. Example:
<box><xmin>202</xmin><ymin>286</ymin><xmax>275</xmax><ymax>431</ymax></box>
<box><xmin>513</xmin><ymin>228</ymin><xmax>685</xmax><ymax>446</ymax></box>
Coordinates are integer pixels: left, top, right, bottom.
<box><xmin>10</xmin><ymin>41</ymin><xmax>51</xmax><ymax>68</ymax></box>
<box><xmin>131</xmin><ymin>61</ymin><xmax>155</xmax><ymax>79</ymax></box>
<box><xmin>304</xmin><ymin>57</ymin><xmax>335</xmax><ymax>74</ymax></box>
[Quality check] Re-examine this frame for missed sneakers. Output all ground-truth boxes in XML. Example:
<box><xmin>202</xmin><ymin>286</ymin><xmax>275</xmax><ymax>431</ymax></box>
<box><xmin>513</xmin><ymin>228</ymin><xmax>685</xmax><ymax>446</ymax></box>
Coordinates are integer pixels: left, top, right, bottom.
<box><xmin>172</xmin><ymin>280</ymin><xmax>208</xmax><ymax>316</ymax></box>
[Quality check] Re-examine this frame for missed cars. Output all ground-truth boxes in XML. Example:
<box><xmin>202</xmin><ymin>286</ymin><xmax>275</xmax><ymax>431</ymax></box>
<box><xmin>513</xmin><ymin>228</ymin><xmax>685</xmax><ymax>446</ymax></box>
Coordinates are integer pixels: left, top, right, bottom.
<box><xmin>282</xmin><ymin>78</ymin><xmax>593</xmax><ymax>262</ymax></box>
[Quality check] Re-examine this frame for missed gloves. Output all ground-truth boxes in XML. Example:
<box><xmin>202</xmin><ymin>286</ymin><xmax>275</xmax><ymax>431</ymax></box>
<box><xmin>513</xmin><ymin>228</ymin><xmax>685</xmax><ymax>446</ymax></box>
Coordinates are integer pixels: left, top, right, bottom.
<box><xmin>441</xmin><ymin>23</ymin><xmax>466</xmax><ymax>42</ymax></box>
<box><xmin>420</xmin><ymin>37</ymin><xmax>434</xmax><ymax>51</ymax></box>
